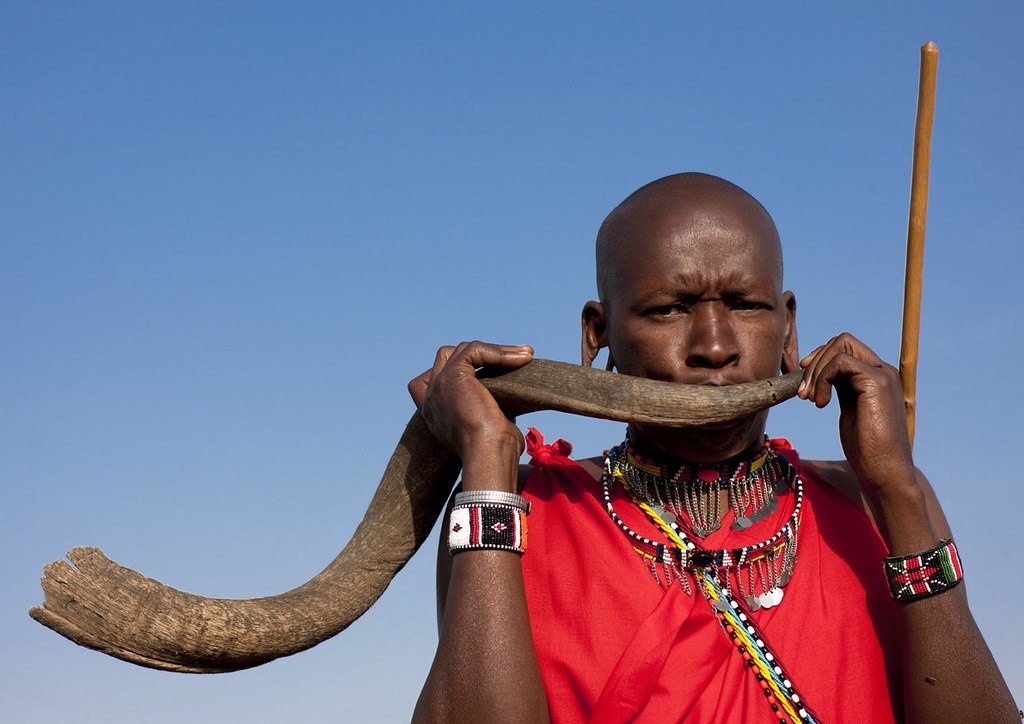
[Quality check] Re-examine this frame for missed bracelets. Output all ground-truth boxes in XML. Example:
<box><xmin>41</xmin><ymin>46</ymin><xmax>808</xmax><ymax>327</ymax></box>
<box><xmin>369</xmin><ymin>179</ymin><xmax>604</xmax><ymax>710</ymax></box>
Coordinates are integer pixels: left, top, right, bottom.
<box><xmin>883</xmin><ymin>539</ymin><xmax>964</xmax><ymax>603</ymax></box>
<box><xmin>447</xmin><ymin>504</ymin><xmax>528</xmax><ymax>557</ymax></box>
<box><xmin>455</xmin><ymin>490</ymin><xmax>531</xmax><ymax>514</ymax></box>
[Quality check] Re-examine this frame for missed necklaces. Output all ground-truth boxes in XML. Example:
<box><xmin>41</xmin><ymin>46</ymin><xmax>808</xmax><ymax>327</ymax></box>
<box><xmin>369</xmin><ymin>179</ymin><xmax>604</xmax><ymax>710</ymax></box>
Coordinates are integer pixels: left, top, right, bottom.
<box><xmin>602</xmin><ymin>430</ymin><xmax>805</xmax><ymax>611</ymax></box>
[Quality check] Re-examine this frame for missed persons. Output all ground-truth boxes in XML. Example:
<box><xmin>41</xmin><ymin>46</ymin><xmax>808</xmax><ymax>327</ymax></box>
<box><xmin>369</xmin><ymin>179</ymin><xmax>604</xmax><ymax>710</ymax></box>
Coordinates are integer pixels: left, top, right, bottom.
<box><xmin>408</xmin><ymin>171</ymin><xmax>1022</xmax><ymax>724</ymax></box>
